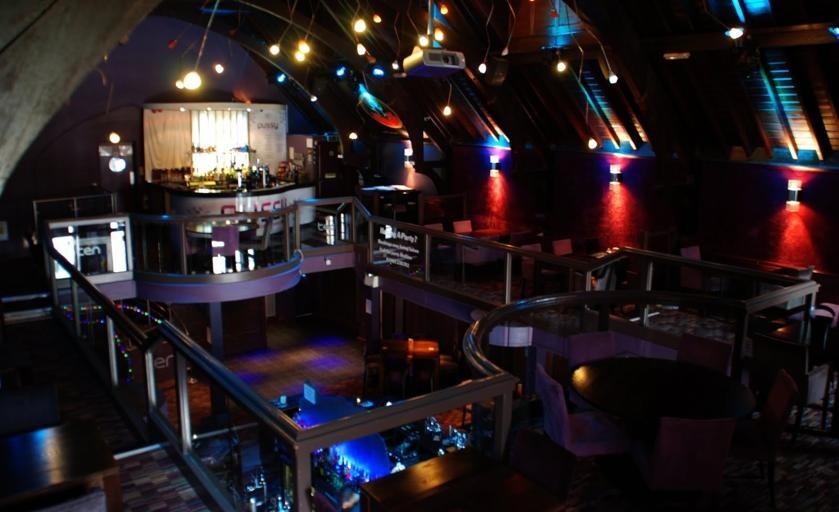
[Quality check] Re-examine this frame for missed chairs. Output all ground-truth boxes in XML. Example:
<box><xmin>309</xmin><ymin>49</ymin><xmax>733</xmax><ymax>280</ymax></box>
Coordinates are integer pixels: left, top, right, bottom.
<box><xmin>423</xmin><ymin>220</ymin><xmax>575</xmax><ymax>300</ymax></box>
<box><xmin>1</xmin><ymin>325</ymin><xmax>61</xmax><ymax>432</ymax></box>
<box><xmin>680</xmin><ymin>245</ymin><xmax>704</xmax><ymax>289</ymax></box>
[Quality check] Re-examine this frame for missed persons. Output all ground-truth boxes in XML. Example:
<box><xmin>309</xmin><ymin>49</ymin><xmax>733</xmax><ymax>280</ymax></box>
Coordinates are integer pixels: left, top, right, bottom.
<box><xmin>202</xmin><ymin>157</ymin><xmax>299</xmax><ymax>190</ymax></box>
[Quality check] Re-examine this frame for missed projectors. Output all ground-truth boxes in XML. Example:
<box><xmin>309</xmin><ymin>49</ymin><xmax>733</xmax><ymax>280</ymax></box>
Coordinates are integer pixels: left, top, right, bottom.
<box><xmin>403</xmin><ymin>49</ymin><xmax>465</xmax><ymax>78</ymax></box>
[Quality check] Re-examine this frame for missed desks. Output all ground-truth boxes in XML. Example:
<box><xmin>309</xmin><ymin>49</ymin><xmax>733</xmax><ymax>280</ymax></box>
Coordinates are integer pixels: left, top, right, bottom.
<box><xmin>0</xmin><ymin>416</ymin><xmax>122</xmax><ymax>510</ymax></box>
